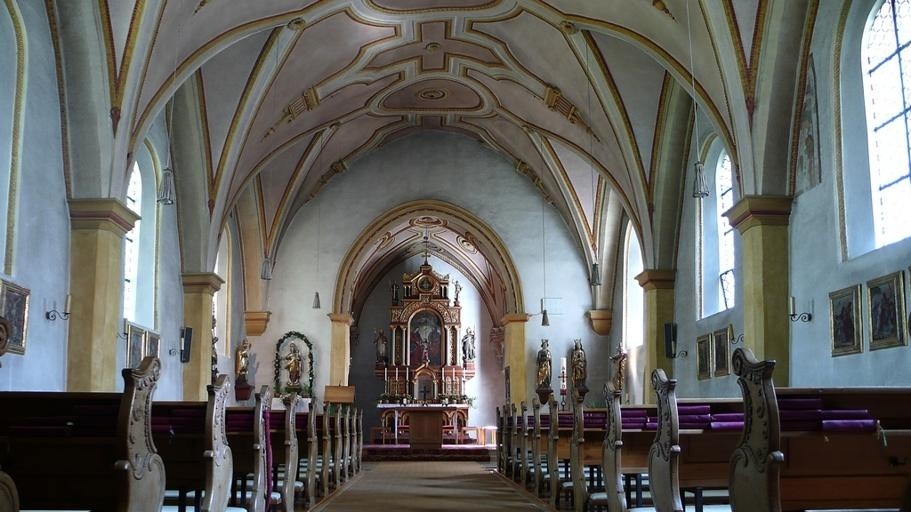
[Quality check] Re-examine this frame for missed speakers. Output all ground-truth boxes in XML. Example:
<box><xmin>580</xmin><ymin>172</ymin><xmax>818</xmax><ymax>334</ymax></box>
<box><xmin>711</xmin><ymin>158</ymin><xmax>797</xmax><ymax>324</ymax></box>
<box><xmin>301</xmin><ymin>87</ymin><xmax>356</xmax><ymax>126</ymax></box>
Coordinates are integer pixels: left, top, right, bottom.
<box><xmin>664</xmin><ymin>322</ymin><xmax>677</xmax><ymax>358</ymax></box>
<box><xmin>181</xmin><ymin>327</ymin><xmax>192</xmax><ymax>361</ymax></box>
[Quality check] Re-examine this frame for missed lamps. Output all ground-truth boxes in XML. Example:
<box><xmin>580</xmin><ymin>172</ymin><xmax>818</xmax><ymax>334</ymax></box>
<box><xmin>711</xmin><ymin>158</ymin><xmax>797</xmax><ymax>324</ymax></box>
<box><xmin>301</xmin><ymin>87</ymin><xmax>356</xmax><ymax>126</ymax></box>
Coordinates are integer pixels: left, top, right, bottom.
<box><xmin>156</xmin><ymin>23</ymin><xmax>180</xmax><ymax>206</ymax></box>
<box><xmin>312</xmin><ymin>129</ymin><xmax>322</xmax><ymax>309</ymax></box>
<box><xmin>540</xmin><ymin>134</ymin><xmax>550</xmax><ymax>327</ymax></box>
<box><xmin>585</xmin><ymin>30</ymin><xmax>601</xmax><ymax>287</ymax></box>
<box><xmin>683</xmin><ymin>0</ymin><xmax>710</xmax><ymax>199</ymax></box>
<box><xmin>261</xmin><ymin>27</ymin><xmax>279</xmax><ymax>281</ymax></box>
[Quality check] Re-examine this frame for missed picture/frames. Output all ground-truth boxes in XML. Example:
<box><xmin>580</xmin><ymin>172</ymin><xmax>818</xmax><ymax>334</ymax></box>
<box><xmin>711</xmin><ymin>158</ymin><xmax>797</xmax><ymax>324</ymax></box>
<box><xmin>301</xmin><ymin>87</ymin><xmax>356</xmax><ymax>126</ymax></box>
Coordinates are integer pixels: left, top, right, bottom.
<box><xmin>145</xmin><ymin>330</ymin><xmax>162</xmax><ymax>358</ymax></box>
<box><xmin>695</xmin><ymin>333</ymin><xmax>712</xmax><ymax>382</ymax></box>
<box><xmin>712</xmin><ymin>327</ymin><xmax>730</xmax><ymax>378</ymax></box>
<box><xmin>866</xmin><ymin>270</ymin><xmax>908</xmax><ymax>352</ymax></box>
<box><xmin>828</xmin><ymin>283</ymin><xmax>864</xmax><ymax>358</ymax></box>
<box><xmin>126</xmin><ymin>322</ymin><xmax>145</xmax><ymax>369</ymax></box>
<box><xmin>0</xmin><ymin>277</ymin><xmax>31</xmax><ymax>355</ymax></box>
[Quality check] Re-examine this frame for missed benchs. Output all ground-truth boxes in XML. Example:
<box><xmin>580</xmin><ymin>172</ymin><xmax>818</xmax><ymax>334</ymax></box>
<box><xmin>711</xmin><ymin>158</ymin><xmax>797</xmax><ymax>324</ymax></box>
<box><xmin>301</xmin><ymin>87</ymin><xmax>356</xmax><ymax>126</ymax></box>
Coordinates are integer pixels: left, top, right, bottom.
<box><xmin>0</xmin><ymin>358</ymin><xmax>362</xmax><ymax>512</ymax></box>
<box><xmin>496</xmin><ymin>349</ymin><xmax>911</xmax><ymax>512</ymax></box>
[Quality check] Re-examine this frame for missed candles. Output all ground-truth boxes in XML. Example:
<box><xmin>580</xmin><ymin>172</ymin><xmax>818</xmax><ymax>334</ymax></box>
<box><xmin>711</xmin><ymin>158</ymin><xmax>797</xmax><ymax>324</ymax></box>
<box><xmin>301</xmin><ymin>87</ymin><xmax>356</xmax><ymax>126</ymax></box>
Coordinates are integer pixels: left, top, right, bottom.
<box><xmin>441</xmin><ymin>367</ymin><xmax>466</xmax><ymax>383</ymax></box>
<box><xmin>790</xmin><ymin>297</ymin><xmax>795</xmax><ymax>314</ymax></box>
<box><xmin>729</xmin><ymin>324</ymin><xmax>733</xmax><ymax>338</ymax></box>
<box><xmin>123</xmin><ymin>319</ymin><xmax>128</xmax><ymax>333</ymax></box>
<box><xmin>559</xmin><ymin>357</ymin><xmax>567</xmax><ymax>395</ymax></box>
<box><xmin>384</xmin><ymin>367</ymin><xmax>409</xmax><ymax>382</ymax></box>
<box><xmin>671</xmin><ymin>341</ymin><xmax>676</xmax><ymax>355</ymax></box>
<box><xmin>65</xmin><ymin>294</ymin><xmax>71</xmax><ymax>313</ymax></box>
<box><xmin>181</xmin><ymin>337</ymin><xmax>184</xmax><ymax>350</ymax></box>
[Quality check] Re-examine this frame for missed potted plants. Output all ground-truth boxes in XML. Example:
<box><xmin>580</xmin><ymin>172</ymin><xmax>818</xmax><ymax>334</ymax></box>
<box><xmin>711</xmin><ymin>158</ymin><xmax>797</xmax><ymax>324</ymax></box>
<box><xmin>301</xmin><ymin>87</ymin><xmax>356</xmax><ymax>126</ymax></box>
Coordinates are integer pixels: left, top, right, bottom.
<box><xmin>376</xmin><ymin>393</ymin><xmax>478</xmax><ymax>404</ymax></box>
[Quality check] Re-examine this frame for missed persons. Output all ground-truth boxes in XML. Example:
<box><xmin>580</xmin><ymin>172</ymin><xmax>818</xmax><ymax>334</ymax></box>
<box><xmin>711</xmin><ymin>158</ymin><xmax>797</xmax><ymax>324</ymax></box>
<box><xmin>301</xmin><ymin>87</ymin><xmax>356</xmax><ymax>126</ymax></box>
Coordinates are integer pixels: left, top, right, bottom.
<box><xmin>428</xmin><ymin>326</ymin><xmax>442</xmax><ymax>366</ymax></box>
<box><xmin>536</xmin><ymin>338</ymin><xmax>553</xmax><ymax>388</ymax></box>
<box><xmin>373</xmin><ymin>329</ymin><xmax>389</xmax><ymax>363</ymax></box>
<box><xmin>609</xmin><ymin>343</ymin><xmax>627</xmax><ymax>393</ymax></box>
<box><xmin>571</xmin><ymin>338</ymin><xmax>588</xmax><ymax>386</ymax></box>
<box><xmin>411</xmin><ymin>326</ymin><xmax>424</xmax><ymax>364</ymax></box>
<box><xmin>285</xmin><ymin>343</ymin><xmax>302</xmax><ymax>382</ymax></box>
<box><xmin>463</xmin><ymin>327</ymin><xmax>477</xmax><ymax>360</ymax></box>
<box><xmin>237</xmin><ymin>337</ymin><xmax>253</xmax><ymax>375</ymax></box>
<box><xmin>421</xmin><ymin>337</ymin><xmax>430</xmax><ymax>363</ymax></box>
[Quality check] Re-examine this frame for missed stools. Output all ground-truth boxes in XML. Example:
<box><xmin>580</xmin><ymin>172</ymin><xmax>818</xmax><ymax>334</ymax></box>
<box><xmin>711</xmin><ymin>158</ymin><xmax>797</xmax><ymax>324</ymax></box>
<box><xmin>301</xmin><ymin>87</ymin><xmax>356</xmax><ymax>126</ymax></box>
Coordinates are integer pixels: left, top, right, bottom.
<box><xmin>370</xmin><ymin>426</ymin><xmax>498</xmax><ymax>445</ymax></box>
